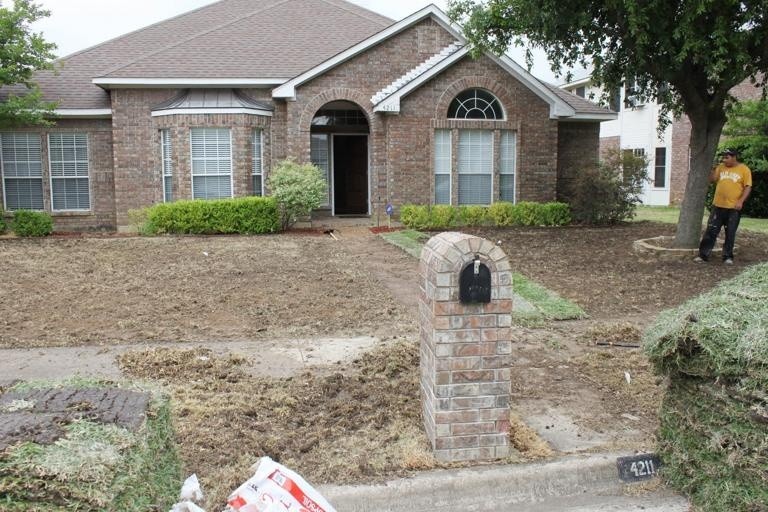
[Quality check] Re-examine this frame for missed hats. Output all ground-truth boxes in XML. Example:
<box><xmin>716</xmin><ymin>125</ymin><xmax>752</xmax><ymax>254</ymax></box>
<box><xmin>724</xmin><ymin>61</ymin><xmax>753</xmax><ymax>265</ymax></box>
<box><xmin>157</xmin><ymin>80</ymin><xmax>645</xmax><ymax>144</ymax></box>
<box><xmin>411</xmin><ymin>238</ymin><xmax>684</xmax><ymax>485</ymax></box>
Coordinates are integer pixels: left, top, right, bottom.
<box><xmin>717</xmin><ymin>148</ymin><xmax>736</xmax><ymax>155</ymax></box>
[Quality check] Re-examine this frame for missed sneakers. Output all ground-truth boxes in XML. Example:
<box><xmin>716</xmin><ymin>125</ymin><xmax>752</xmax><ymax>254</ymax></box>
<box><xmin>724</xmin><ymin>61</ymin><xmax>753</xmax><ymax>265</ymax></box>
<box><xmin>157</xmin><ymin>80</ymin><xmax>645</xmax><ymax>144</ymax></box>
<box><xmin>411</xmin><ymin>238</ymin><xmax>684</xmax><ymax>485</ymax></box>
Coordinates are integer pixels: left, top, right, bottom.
<box><xmin>723</xmin><ymin>259</ymin><xmax>732</xmax><ymax>265</ymax></box>
<box><xmin>692</xmin><ymin>257</ymin><xmax>707</xmax><ymax>263</ymax></box>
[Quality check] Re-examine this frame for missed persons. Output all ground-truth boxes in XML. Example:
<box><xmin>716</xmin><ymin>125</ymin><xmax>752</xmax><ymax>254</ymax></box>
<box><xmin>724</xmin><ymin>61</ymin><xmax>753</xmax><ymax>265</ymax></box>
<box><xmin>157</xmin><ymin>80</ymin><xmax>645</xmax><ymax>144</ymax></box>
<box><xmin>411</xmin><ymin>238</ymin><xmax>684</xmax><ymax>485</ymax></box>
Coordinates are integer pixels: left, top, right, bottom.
<box><xmin>692</xmin><ymin>147</ymin><xmax>753</xmax><ymax>265</ymax></box>
